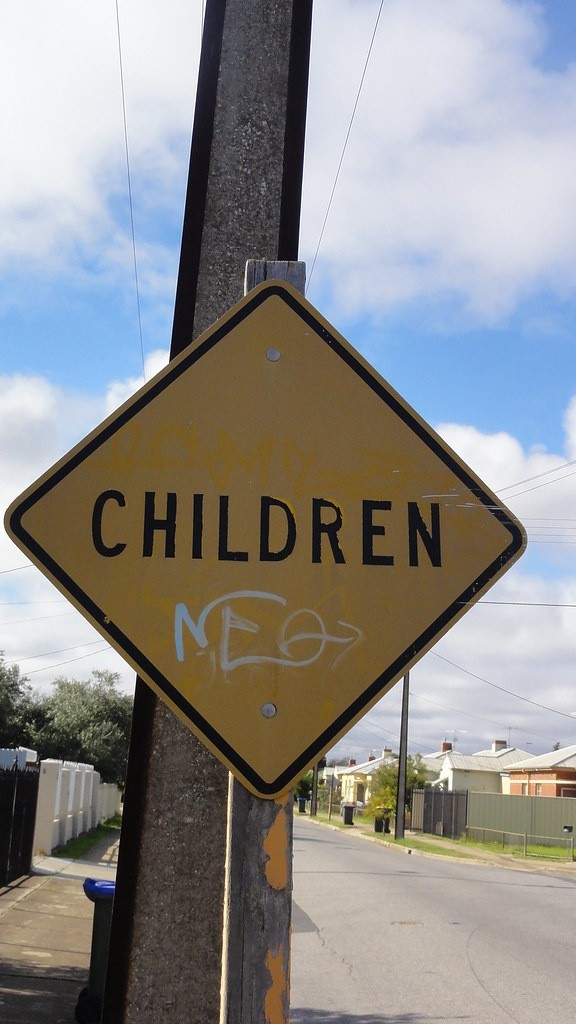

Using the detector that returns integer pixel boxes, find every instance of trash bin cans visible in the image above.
[374,807,394,834]
[343,805,355,825]
[74,877,116,1024]
[297,797,306,813]
